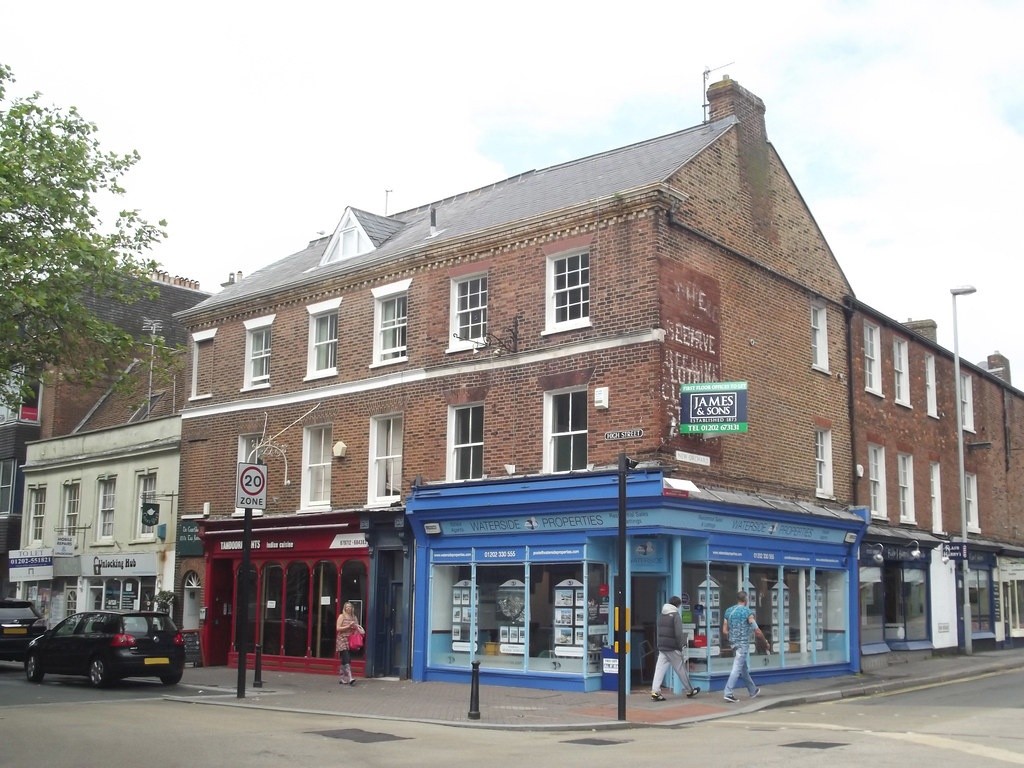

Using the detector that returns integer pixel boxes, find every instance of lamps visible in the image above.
[452,318,521,357]
[933,545,953,565]
[865,541,884,564]
[906,540,922,558]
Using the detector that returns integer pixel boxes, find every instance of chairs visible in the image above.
[91,622,104,633]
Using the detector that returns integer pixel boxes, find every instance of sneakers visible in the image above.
[687,687,700,697]
[724,696,740,703]
[650,692,666,701]
[750,688,760,698]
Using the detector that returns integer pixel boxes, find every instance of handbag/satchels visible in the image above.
[349,629,363,650]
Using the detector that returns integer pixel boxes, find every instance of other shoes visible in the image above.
[350,679,355,685]
[339,680,348,684]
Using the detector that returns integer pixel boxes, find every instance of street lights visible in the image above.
[950,283,979,657]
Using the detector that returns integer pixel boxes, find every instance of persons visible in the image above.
[723,592,770,702]
[336,602,365,685]
[651,596,701,701]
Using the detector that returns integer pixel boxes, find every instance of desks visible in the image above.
[548,648,606,673]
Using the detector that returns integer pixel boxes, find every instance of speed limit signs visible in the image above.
[240,466,266,497]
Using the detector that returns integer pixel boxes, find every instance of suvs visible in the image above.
[24,608,187,690]
[0,597,49,663]
